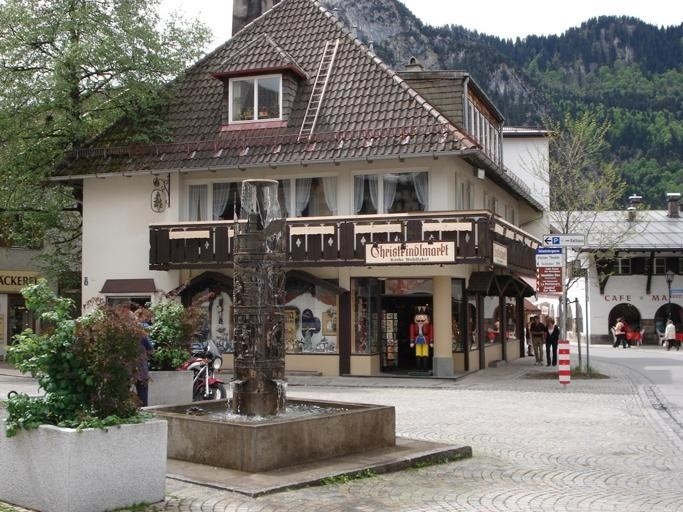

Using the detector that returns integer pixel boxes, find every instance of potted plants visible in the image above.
[0,278,168,512]
[129,299,208,406]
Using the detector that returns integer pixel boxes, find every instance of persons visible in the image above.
[664,319,680,351]
[612,317,629,348]
[526,315,560,366]
[127,309,152,406]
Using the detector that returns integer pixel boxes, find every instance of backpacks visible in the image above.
[620,326,624,332]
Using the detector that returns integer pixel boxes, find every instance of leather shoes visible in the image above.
[546,363,556,367]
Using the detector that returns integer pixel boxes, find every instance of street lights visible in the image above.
[665,269,675,321]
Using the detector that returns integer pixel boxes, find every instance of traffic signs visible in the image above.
[542,233,585,248]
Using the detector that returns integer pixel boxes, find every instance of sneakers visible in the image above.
[527,352,534,356]
[535,361,543,365]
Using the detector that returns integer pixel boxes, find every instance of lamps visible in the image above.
[151,173,170,212]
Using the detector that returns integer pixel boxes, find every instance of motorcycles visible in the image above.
[175,341,228,403]
